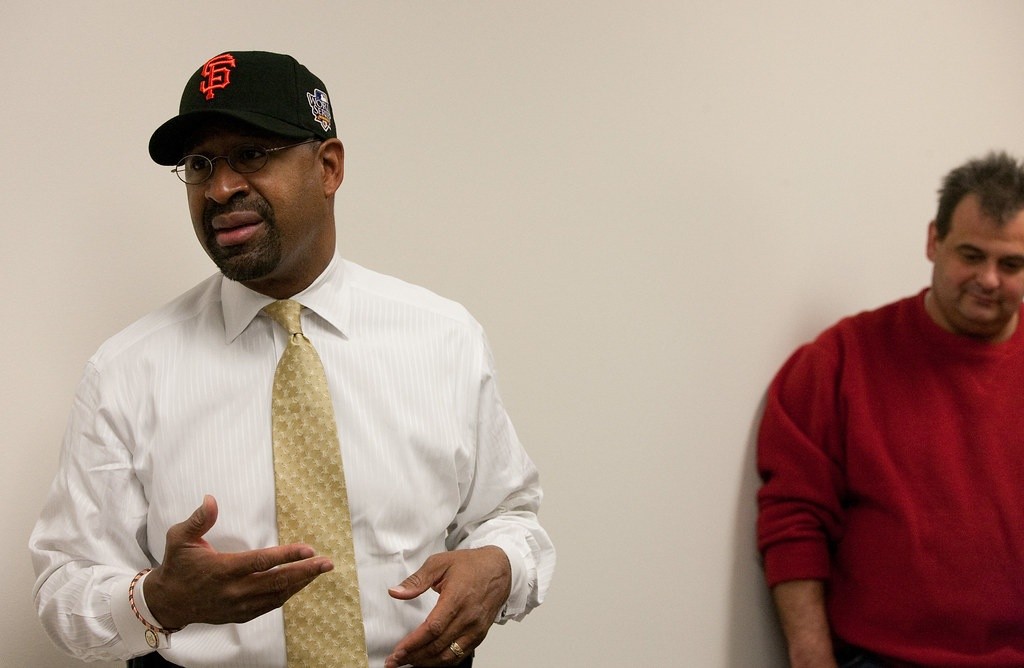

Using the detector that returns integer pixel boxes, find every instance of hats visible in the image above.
[149,51,337,167]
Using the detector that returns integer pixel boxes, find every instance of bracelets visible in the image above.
[129,570,187,635]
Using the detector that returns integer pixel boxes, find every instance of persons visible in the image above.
[27,51,555,668]
[756,154,1024,668]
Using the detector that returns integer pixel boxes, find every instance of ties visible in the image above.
[258,301,369,668]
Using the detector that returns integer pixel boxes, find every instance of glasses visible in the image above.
[171,138,318,186]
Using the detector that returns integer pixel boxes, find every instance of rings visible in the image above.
[447,642,463,658]
